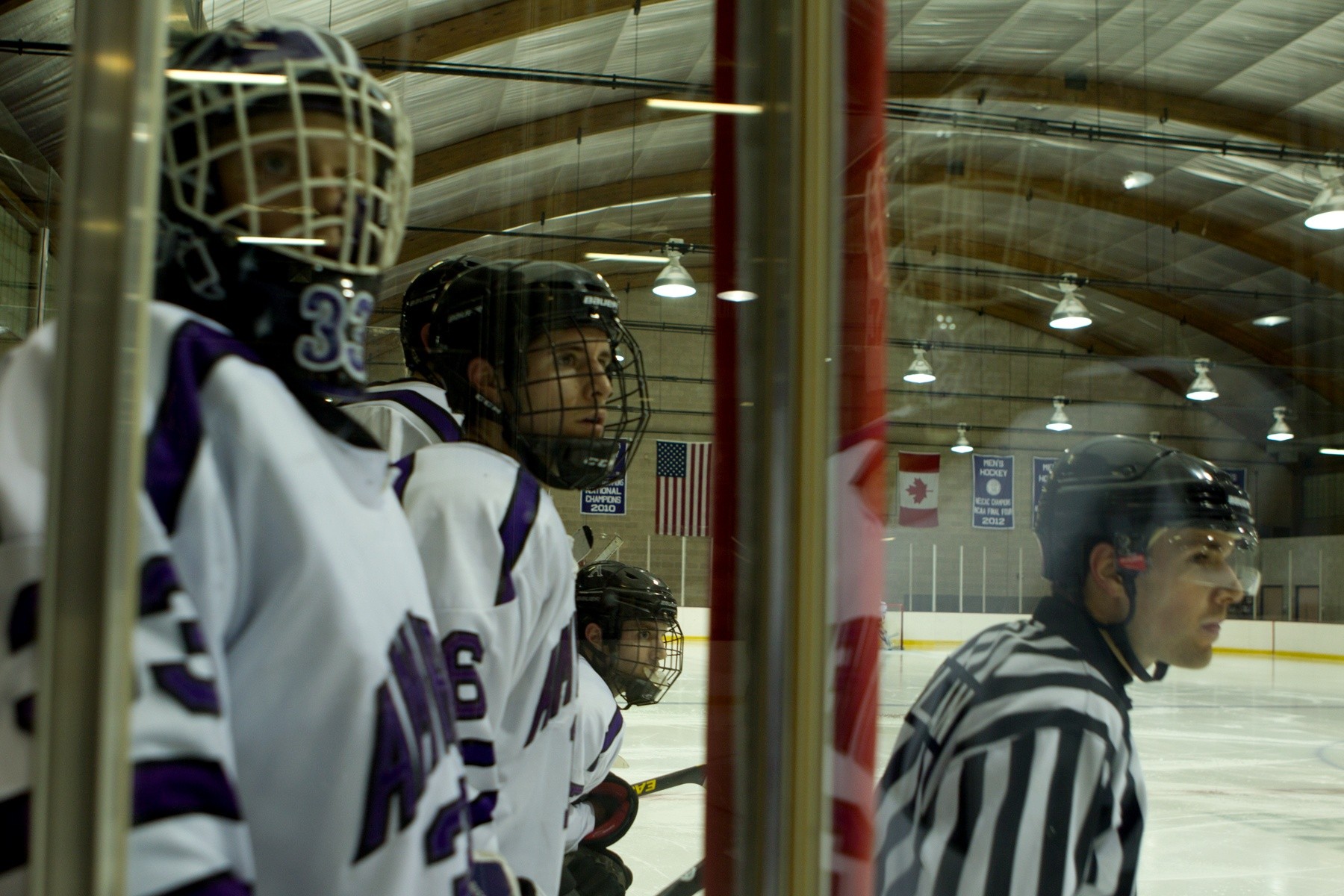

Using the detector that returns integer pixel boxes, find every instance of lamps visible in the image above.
[1046,395,1073,431]
[1266,406,1294,441]
[1305,154,1344,230]
[951,423,973,452]
[903,340,935,383]
[652,237,696,298]
[1185,357,1219,401]
[1049,273,1092,329]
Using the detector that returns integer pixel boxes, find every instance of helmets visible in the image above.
[399,260,485,387]
[574,559,684,710]
[1033,436,1256,579]
[149,17,415,388]
[429,254,652,493]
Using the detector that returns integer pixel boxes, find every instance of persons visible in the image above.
[324,256,686,896]
[871,437,1261,896]
[0,16,473,896]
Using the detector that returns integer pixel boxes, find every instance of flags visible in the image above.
[654,439,712,537]
[897,450,940,529]
